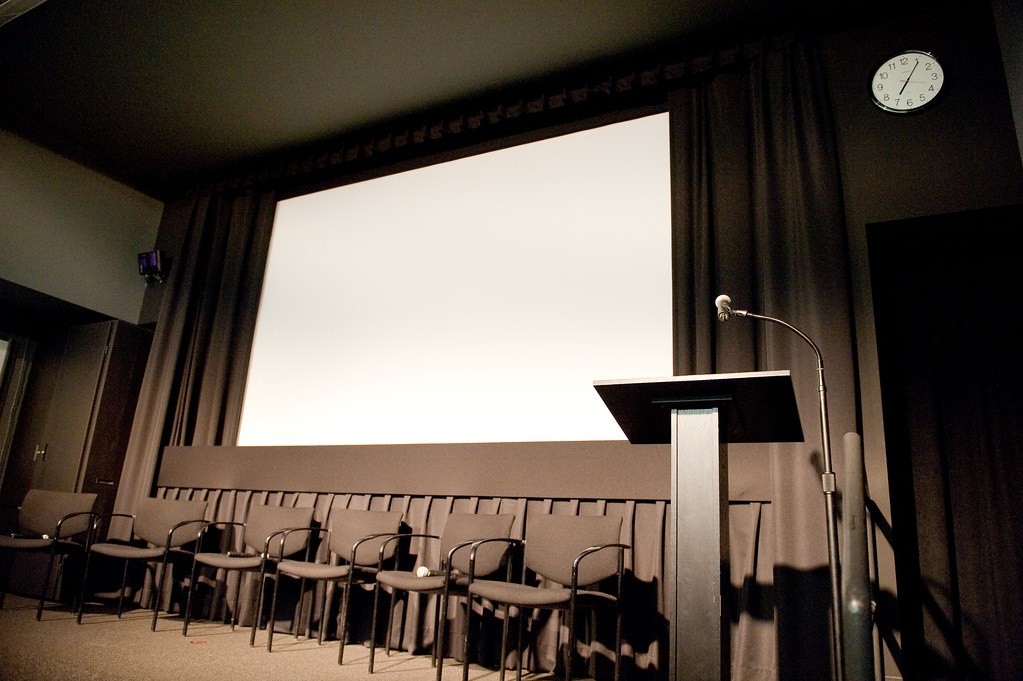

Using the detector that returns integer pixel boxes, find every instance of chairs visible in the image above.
[0,490,98,620]
[366,512,514,680]
[182,504,313,644]
[462,513,630,681]
[76,496,208,630]
[266,507,404,662]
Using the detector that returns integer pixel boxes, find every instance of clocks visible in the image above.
[866,46,948,115]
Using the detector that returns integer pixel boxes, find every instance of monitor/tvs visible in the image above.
[137,250,160,275]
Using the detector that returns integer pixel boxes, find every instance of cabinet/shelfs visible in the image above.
[1,319,154,602]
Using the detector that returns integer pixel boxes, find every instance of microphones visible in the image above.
[715,294,731,321]
